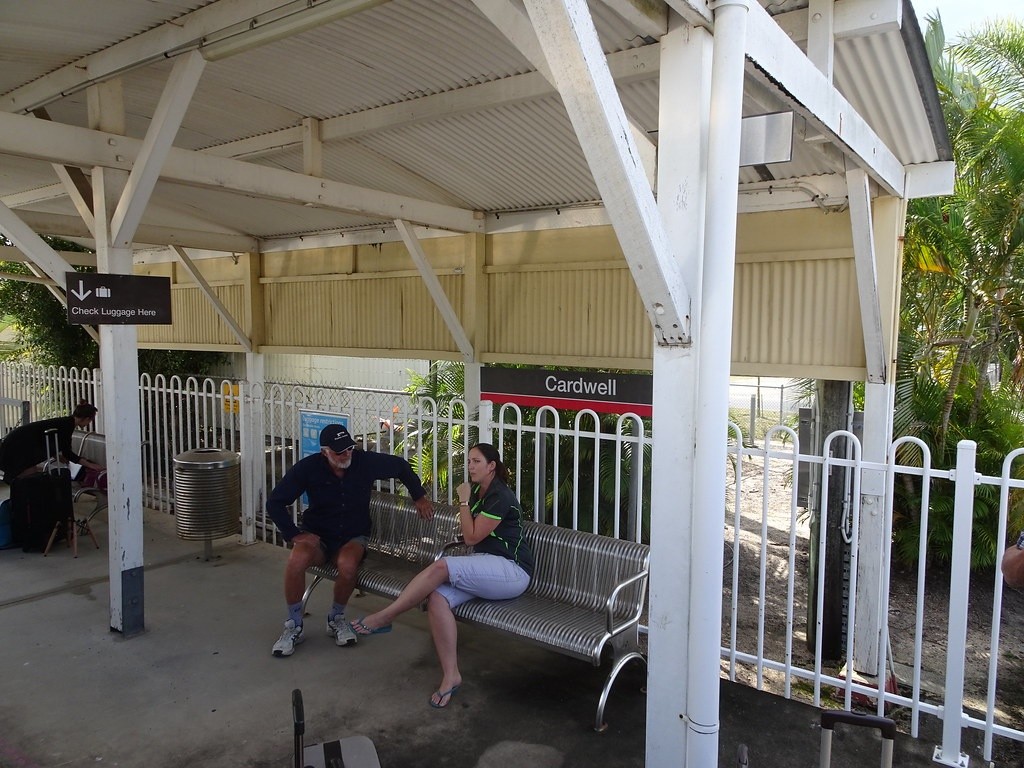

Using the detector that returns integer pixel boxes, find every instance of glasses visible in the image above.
[325,446,354,455]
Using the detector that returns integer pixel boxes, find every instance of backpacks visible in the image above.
[0,499,11,550]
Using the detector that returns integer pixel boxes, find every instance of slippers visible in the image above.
[430,684,459,708]
[348,620,396,637]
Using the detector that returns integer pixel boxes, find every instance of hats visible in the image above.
[319,424,358,453]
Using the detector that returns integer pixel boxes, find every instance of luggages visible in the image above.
[10,428,73,552]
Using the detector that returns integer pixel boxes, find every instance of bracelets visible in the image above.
[459,501,470,507]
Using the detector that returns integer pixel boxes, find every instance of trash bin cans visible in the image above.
[174,448,242,542]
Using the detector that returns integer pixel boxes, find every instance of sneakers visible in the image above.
[272,618,306,656]
[327,614,357,646]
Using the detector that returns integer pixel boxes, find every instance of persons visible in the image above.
[0,403,104,486]
[348,444,532,709]
[265,425,434,659]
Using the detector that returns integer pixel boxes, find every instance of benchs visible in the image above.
[57,429,150,536]
[291,488,651,734]
